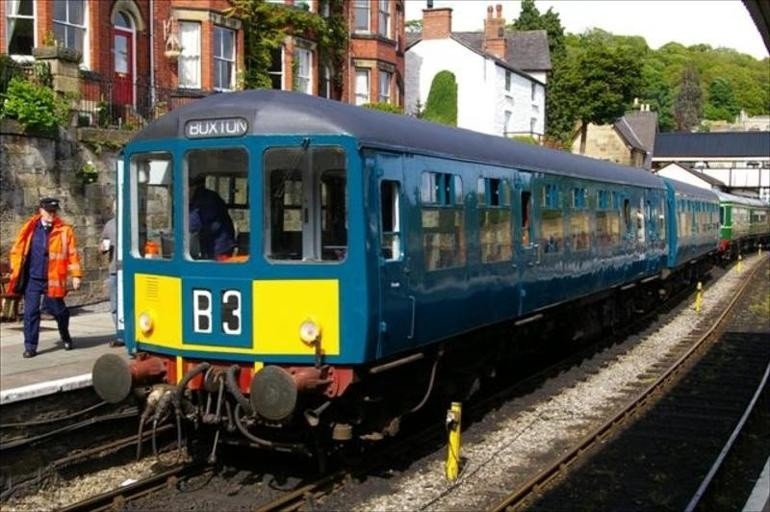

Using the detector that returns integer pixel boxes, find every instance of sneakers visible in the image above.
[24,350,36,357]
[65,341,71,348]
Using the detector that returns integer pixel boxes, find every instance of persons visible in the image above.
[97,199,124,346]
[4,197,82,358]
[189,177,238,259]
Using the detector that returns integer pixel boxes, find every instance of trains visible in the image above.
[92,89,770,474]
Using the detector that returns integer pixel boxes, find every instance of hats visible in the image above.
[40,198,60,211]
